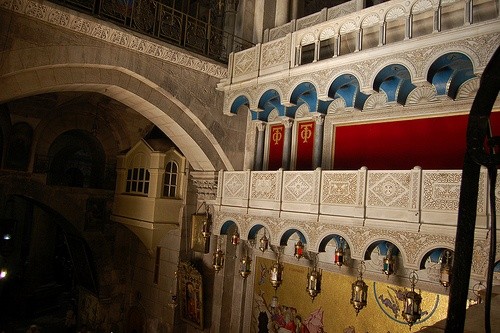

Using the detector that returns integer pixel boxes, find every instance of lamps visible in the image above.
[200,219,488,329]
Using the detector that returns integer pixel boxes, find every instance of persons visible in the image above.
[26,324,40,333]
[77,324,91,333]
[63,304,74,333]
